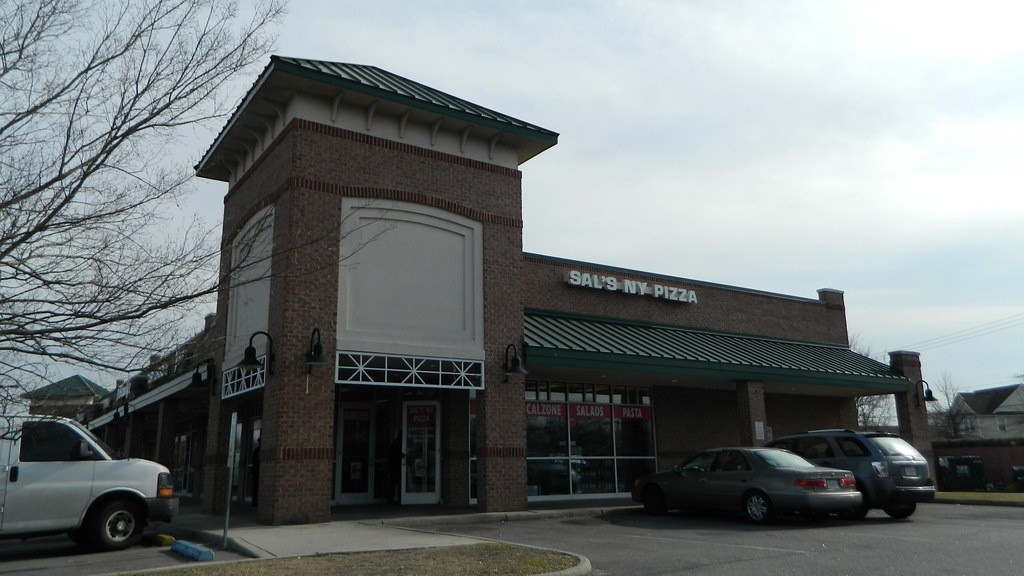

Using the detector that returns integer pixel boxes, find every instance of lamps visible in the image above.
[307,328,329,374]
[235,331,275,375]
[503,344,528,376]
[915,380,938,408]
[186,358,217,394]
[111,401,130,426]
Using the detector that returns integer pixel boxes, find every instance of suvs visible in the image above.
[762,427,934,518]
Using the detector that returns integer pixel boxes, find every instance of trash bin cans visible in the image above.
[938,454,984,489]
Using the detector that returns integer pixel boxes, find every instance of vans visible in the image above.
[0,412,180,551]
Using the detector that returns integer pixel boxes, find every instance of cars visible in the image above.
[630,448,864,526]
